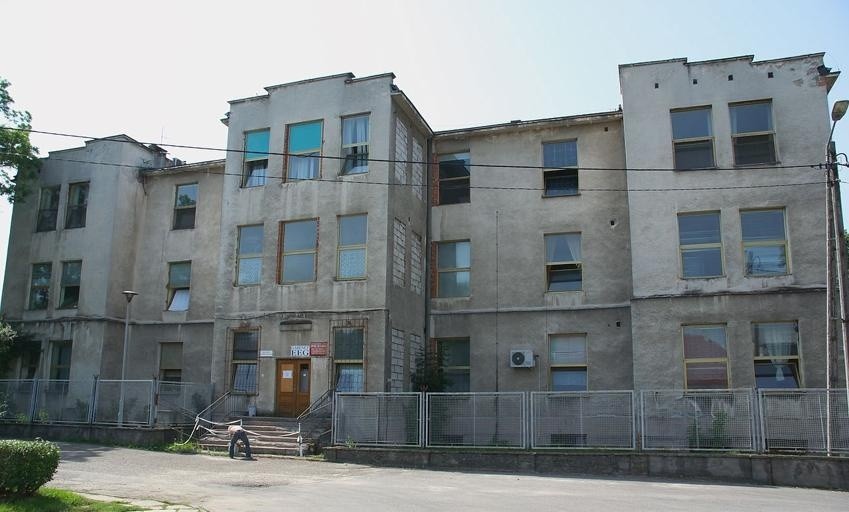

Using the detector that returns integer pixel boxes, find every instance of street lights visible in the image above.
[117,290,140,428]
[818,97,849,457]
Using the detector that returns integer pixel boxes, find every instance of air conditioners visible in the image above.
[173,157,183,165]
[509,349,535,367]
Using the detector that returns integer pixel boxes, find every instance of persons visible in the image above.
[227,425,260,459]
